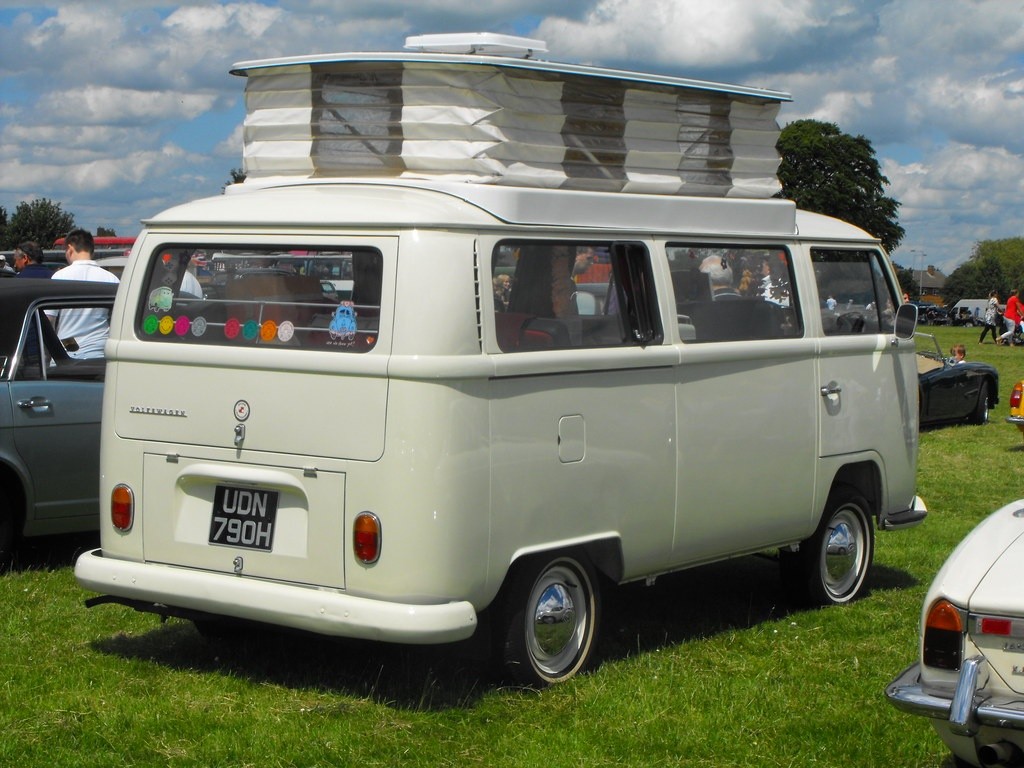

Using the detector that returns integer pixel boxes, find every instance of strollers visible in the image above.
[999,312,1023,346]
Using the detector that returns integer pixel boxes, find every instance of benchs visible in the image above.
[491,312,574,350]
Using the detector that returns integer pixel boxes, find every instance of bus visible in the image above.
[212,251,354,302]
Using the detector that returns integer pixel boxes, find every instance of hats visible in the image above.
[0,254,6,260]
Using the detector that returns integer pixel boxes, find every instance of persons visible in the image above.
[0,254,14,272]
[866,301,876,310]
[978,290,1004,343]
[494,274,510,300]
[700,255,743,302]
[872,289,910,324]
[996,289,1024,347]
[570,246,596,283]
[178,270,202,299]
[826,294,837,312]
[951,344,965,363]
[757,255,789,307]
[43,229,120,382]
[926,306,973,327]
[14,241,57,365]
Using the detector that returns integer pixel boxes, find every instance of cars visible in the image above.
[914,331,1000,426]
[0,278,210,576]
[882,497,1024,768]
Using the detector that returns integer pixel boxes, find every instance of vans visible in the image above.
[946,300,990,326]
[75,32,929,694]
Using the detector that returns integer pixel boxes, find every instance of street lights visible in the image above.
[911,249,924,295]
[912,254,928,277]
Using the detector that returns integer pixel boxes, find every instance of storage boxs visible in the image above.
[219,272,323,336]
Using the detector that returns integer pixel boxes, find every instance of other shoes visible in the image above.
[996,337,1002,345]
[978,341,983,345]
[1010,343,1015,347]
